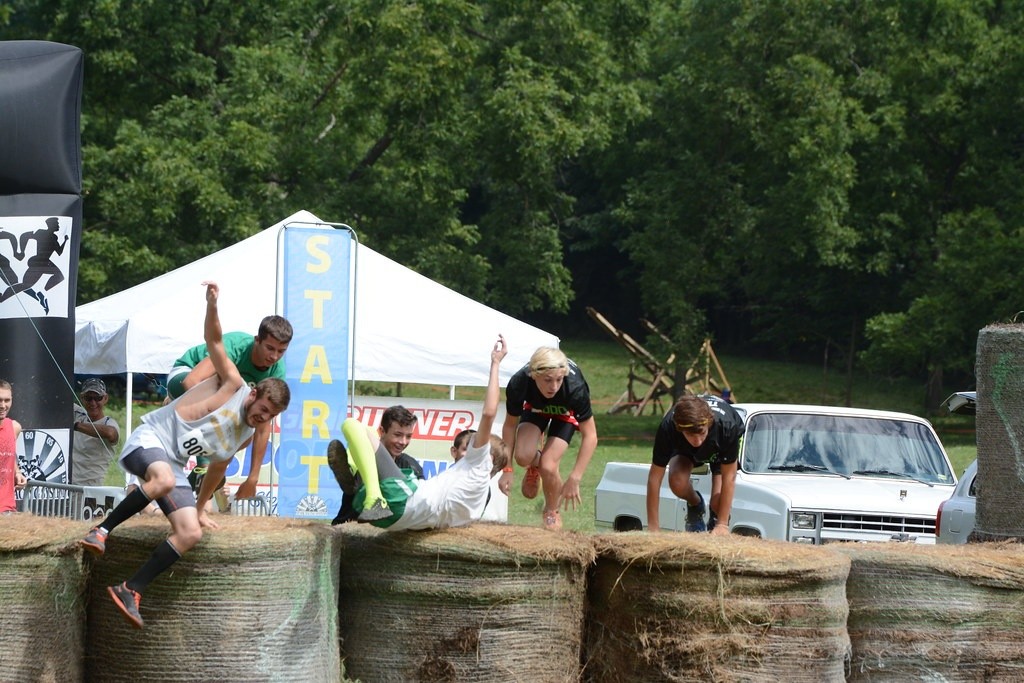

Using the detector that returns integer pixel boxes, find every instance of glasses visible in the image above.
[83,394,104,402]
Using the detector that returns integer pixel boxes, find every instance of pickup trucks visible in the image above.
[591,404,957,552]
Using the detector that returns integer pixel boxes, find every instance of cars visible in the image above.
[935,392,980,545]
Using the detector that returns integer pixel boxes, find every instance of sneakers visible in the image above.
[684,491,706,533]
[358,495,392,521]
[81,526,108,556]
[522,450,543,498]
[541,506,561,531]
[107,581,146,630]
[703,505,732,530]
[327,440,352,490]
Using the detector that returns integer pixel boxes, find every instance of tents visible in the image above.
[72,207,563,488]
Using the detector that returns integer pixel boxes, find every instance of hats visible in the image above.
[80,379,106,396]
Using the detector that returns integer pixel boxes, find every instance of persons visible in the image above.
[498,346,597,530]
[646,392,745,535]
[79,279,291,626]
[0,378,26,516]
[163,313,292,506]
[376,403,425,479]
[72,376,119,521]
[447,429,477,470]
[329,328,507,532]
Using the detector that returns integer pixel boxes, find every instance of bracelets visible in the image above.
[503,468,513,472]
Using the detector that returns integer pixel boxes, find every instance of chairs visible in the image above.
[232,496,270,516]
